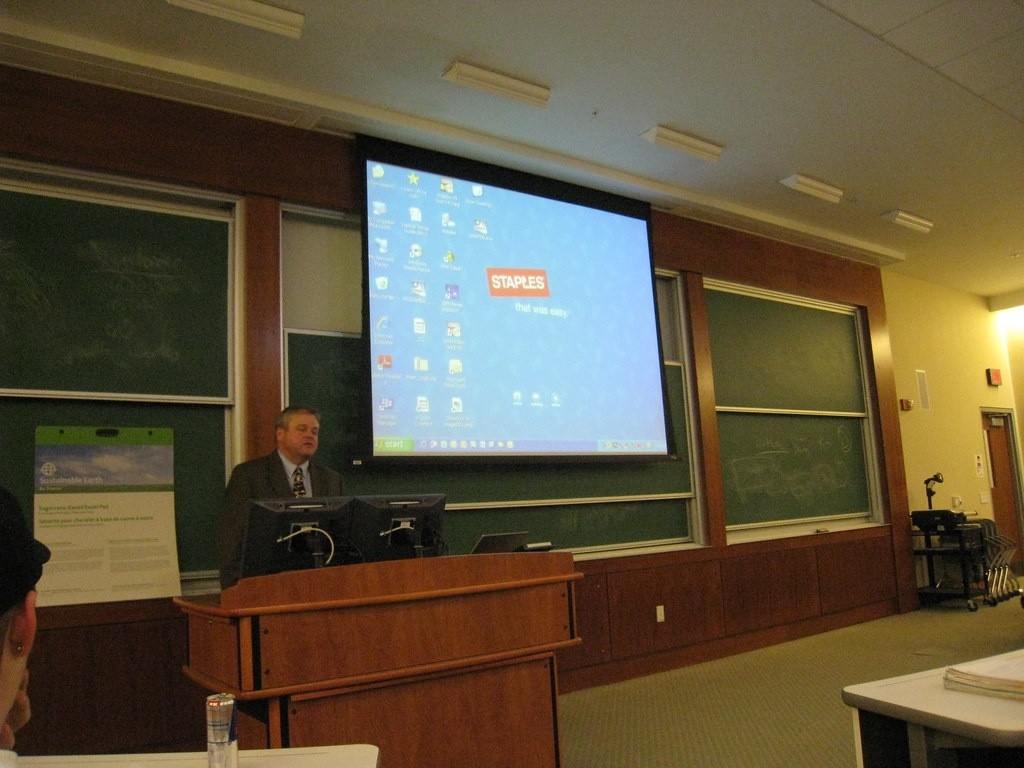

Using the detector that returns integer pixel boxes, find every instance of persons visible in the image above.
[0,487,51,768]
[216,404,354,592]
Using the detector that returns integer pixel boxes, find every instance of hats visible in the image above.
[0,486,51,615]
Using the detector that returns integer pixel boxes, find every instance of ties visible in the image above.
[293,467,307,499]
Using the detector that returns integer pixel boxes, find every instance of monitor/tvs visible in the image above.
[239,496,353,580]
[346,493,446,565]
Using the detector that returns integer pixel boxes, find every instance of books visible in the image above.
[944,649,1024,700]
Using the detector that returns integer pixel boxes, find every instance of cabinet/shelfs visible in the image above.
[912,524,990,611]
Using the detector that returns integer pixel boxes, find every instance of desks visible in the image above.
[19,741,382,768]
[842,648,1024,768]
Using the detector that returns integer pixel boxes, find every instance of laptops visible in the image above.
[468,531,531,554]
